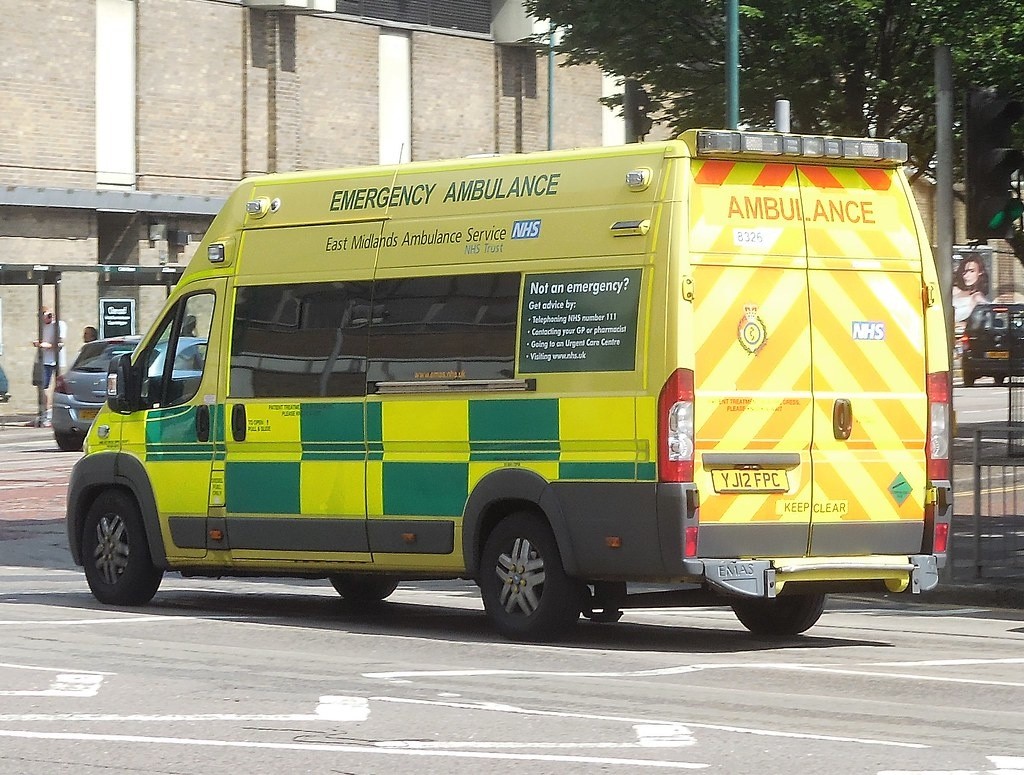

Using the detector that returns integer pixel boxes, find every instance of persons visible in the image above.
[82,325,99,342]
[26,306,68,429]
[182,316,200,338]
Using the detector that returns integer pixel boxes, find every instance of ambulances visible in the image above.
[65,98,955,644]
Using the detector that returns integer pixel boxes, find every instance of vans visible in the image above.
[961,303,1024,387]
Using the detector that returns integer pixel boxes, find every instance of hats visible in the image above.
[42,305,52,316]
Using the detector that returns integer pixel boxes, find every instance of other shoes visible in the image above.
[44,421,52,427]
[24,421,35,427]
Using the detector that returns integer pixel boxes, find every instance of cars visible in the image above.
[51,335,211,451]
